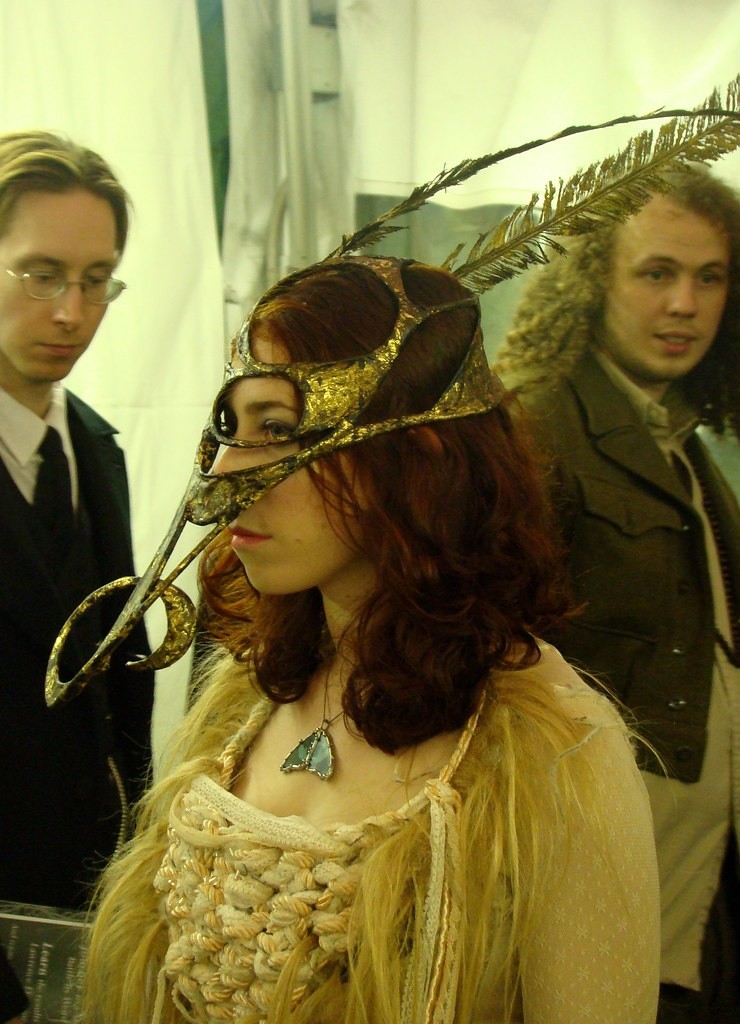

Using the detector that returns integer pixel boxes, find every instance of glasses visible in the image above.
[0,261,127,304]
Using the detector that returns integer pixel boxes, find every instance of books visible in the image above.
[0,899,102,1024]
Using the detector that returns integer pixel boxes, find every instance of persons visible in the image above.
[486,154,740,1024]
[0,129,155,1024]
[79,254,660,1024]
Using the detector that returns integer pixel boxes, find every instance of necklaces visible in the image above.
[278,657,370,781]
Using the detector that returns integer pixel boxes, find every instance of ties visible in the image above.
[32,426,74,570]
